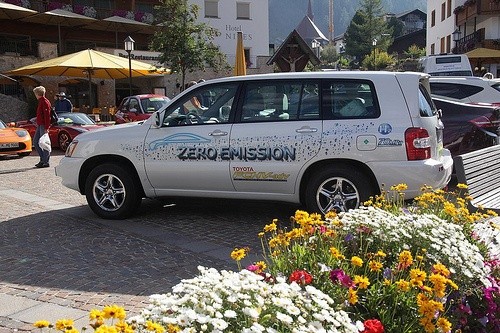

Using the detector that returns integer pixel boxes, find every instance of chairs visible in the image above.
[73,105,115,121]
[246,93,268,120]
[275,94,290,119]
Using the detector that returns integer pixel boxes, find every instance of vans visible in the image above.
[423,53,473,77]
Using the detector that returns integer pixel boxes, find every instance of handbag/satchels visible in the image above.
[38,133,51,152]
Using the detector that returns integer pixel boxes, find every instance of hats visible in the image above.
[58,92,67,96]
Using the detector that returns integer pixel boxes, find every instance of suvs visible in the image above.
[54,72,454,222]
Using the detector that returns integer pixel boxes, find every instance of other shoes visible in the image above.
[35,162,49,168]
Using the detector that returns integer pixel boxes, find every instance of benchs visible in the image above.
[453,145,500,216]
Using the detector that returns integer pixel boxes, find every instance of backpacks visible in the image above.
[40,99,58,123]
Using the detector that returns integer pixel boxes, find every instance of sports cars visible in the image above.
[0,119,32,156]
[15,112,113,153]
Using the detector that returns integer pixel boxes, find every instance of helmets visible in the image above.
[481,67,484,71]
[475,67,478,71]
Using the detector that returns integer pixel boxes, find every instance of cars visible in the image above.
[112,94,180,125]
[290,76,500,191]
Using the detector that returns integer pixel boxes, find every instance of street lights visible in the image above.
[339,43,342,71]
[372,38,378,71]
[123,36,136,96]
[316,42,321,67]
[311,39,318,72]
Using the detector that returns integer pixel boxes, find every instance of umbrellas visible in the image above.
[0,49,177,109]
[234,32,246,76]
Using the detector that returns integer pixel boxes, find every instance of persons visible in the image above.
[180,79,212,115]
[53,92,73,113]
[33,86,52,168]
[72,104,118,121]
